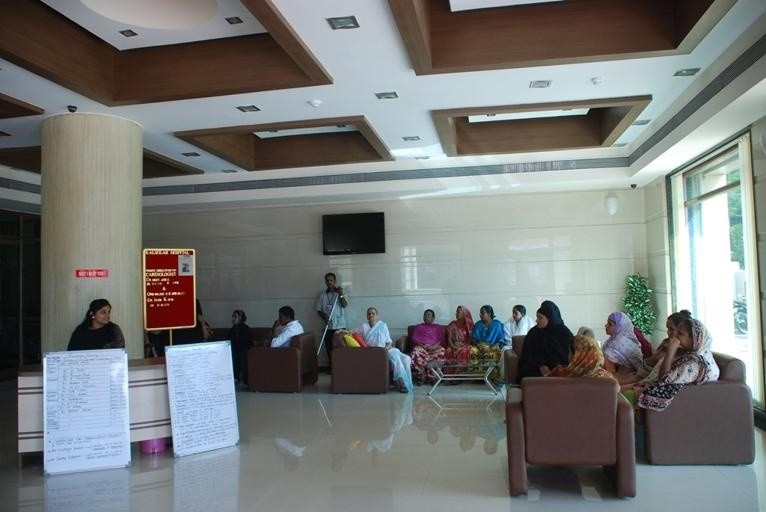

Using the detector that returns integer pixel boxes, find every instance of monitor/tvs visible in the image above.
[321,212,385,256]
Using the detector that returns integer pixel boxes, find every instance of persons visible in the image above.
[518,302,612,379]
[601,310,719,417]
[410,301,533,384]
[266,306,305,348]
[335,307,414,392]
[68,299,125,349]
[412,392,507,454]
[315,272,348,373]
[146,297,250,386]
[265,432,394,474]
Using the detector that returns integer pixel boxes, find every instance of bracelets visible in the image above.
[339,295,344,299]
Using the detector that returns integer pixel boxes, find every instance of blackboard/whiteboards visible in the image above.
[165,340,240,458]
[42,348,131,476]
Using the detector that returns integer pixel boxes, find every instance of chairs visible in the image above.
[330,329,399,394]
[506,376,637,497]
[247,332,318,392]
[504,335,526,393]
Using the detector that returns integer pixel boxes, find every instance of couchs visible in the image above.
[396,324,448,381]
[154,328,264,381]
[643,353,755,465]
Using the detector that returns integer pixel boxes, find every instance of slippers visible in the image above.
[399,386,408,393]
